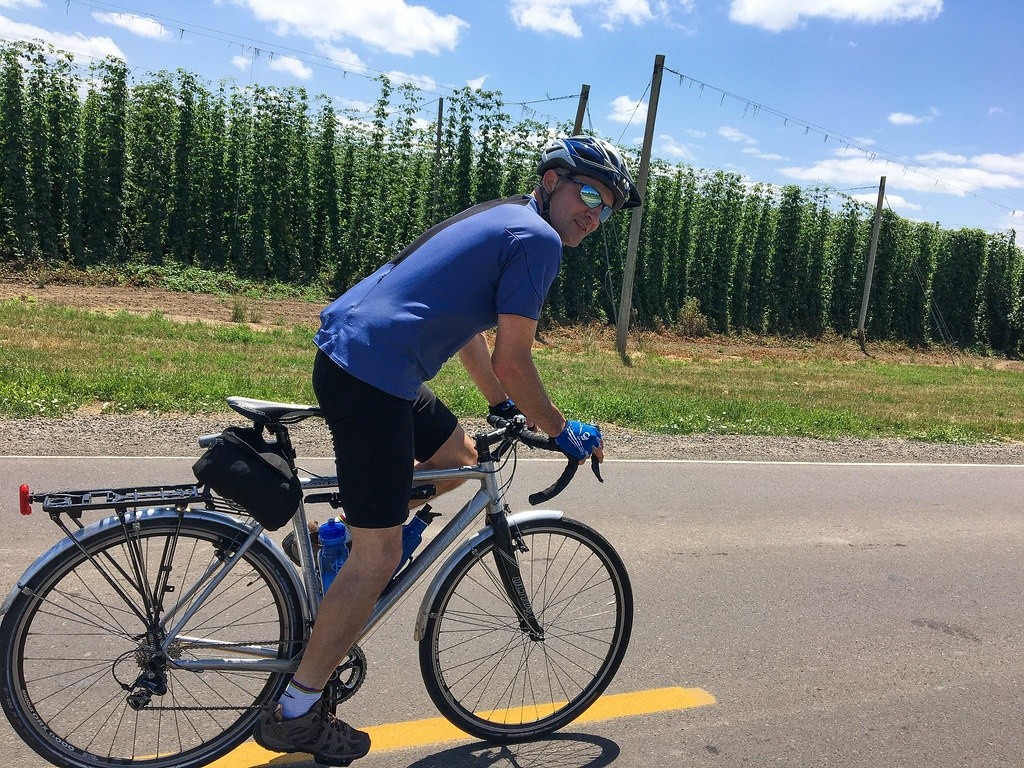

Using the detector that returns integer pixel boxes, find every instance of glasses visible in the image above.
[556,173,613,224]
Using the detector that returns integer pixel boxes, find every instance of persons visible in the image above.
[251,134,642,761]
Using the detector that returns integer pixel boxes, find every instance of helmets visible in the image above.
[536,136,642,211]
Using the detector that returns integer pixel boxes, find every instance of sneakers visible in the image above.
[252,698,371,760]
[281,520,353,570]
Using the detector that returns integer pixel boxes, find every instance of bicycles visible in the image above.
[0,408,633,768]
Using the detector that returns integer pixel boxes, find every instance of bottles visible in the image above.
[317,518,349,597]
[387,504,442,582]
